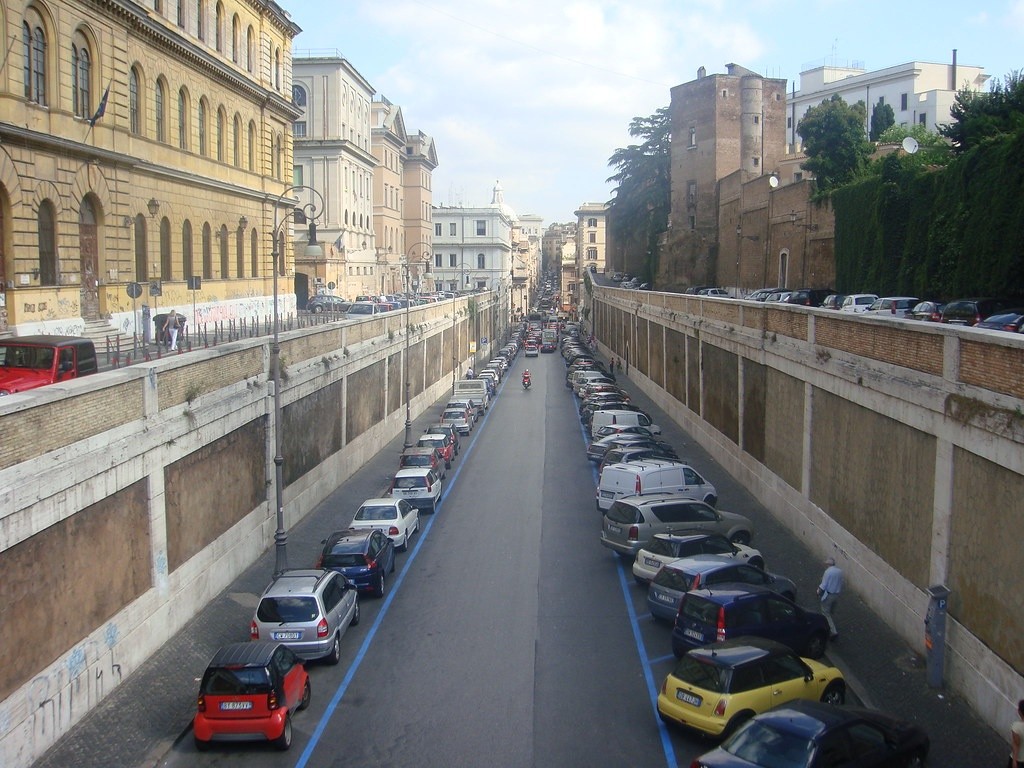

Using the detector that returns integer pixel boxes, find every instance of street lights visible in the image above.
[271,185,328,582]
[472,270,488,379]
[453,262,472,395]
[402,242,434,452]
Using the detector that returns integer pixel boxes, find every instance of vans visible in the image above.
[596,456,718,517]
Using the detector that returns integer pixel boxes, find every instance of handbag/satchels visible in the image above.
[174,316,179,328]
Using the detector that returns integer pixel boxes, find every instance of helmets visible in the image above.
[525,369,529,372]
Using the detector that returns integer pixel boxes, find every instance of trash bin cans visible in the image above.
[473,374,477,380]
[153,313,187,345]
[591,267,596,273]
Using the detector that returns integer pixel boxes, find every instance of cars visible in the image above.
[904,300,947,322]
[818,294,847,310]
[745,287,794,305]
[0,334,98,397]
[972,307,1024,334]
[388,468,442,514]
[836,293,879,313]
[646,553,799,626]
[437,275,682,483]
[399,446,446,480]
[316,529,395,597]
[685,286,730,298]
[632,529,765,589]
[344,301,381,320]
[348,498,419,552]
[423,422,462,457]
[356,288,480,313]
[863,296,920,319]
[688,697,933,768]
[415,433,455,470]
[656,634,846,745]
[191,639,312,752]
[611,271,651,291]
[670,578,830,662]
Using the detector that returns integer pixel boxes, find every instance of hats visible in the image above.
[825,559,834,564]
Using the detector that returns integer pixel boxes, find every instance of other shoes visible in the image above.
[829,633,838,642]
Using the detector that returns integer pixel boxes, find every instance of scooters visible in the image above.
[522,372,531,390]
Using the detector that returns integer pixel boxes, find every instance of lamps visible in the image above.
[737,225,759,242]
[216,216,248,238]
[789,209,819,232]
[375,246,393,257]
[350,241,366,254]
[125,197,160,228]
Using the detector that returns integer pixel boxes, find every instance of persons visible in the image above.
[586,334,599,354]
[1009,699,1024,768]
[521,368,531,386]
[465,366,473,380]
[816,558,844,636]
[580,318,587,336]
[610,356,615,374]
[616,357,623,374]
[162,310,181,351]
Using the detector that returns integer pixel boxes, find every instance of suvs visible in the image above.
[250,568,360,666]
[306,294,352,314]
[939,296,1008,326]
[788,287,831,307]
[599,492,755,560]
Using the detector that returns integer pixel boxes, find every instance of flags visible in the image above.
[90,84,110,127]
